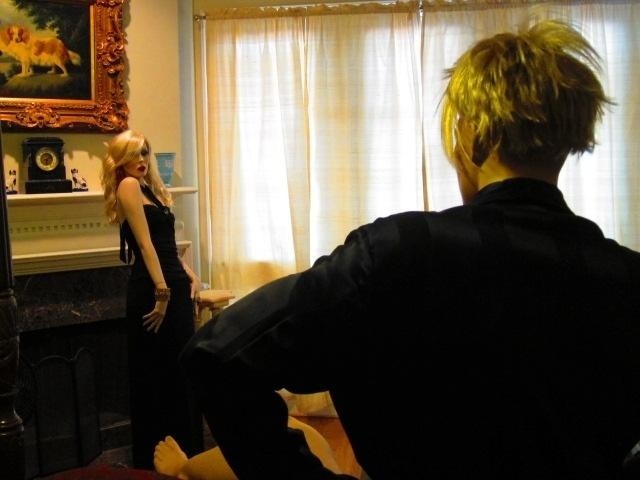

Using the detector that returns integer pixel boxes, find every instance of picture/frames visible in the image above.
[0,0,129,134]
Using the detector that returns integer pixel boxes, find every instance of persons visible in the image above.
[102,129,201,470]
[154,415,360,480]
[180,4,640,480]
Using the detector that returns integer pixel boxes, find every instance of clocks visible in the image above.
[22,136,72,194]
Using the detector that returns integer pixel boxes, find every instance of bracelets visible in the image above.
[154,288,171,301]
